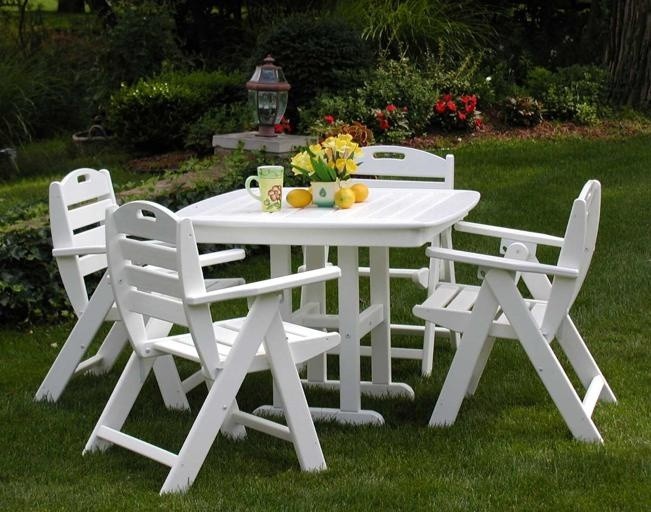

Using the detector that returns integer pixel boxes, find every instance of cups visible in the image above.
[245,164,285,213]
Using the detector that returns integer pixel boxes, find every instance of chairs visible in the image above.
[33,166,246,406]
[298,144,454,379]
[83,198,342,495]
[411,180,617,447]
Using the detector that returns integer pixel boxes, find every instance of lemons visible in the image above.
[334,188,356,209]
[286,189,312,209]
[351,183,368,202]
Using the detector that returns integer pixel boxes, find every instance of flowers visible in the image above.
[288,131,365,182]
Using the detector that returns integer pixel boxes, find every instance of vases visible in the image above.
[310,180,345,206]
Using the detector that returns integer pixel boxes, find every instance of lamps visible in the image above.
[248,54,292,136]
